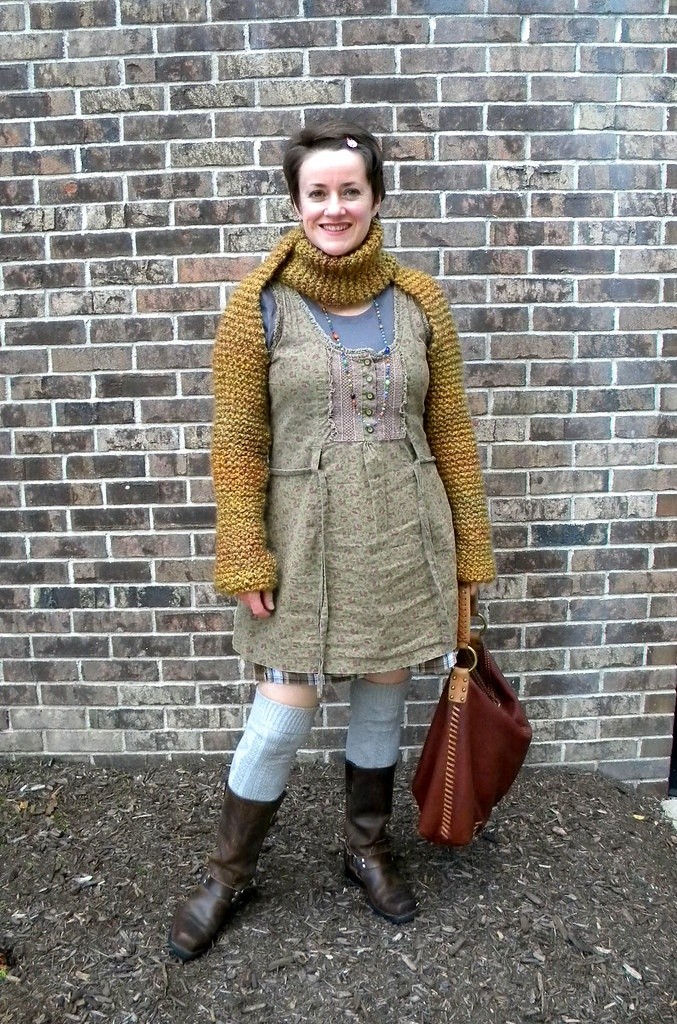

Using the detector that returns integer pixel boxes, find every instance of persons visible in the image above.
[167,117,498,959]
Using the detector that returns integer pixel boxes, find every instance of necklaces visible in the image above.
[322,299,391,427]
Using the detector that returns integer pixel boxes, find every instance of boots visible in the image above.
[169,781,287,961]
[344,758,420,924]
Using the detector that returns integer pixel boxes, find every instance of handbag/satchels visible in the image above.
[413,583,531,848]
